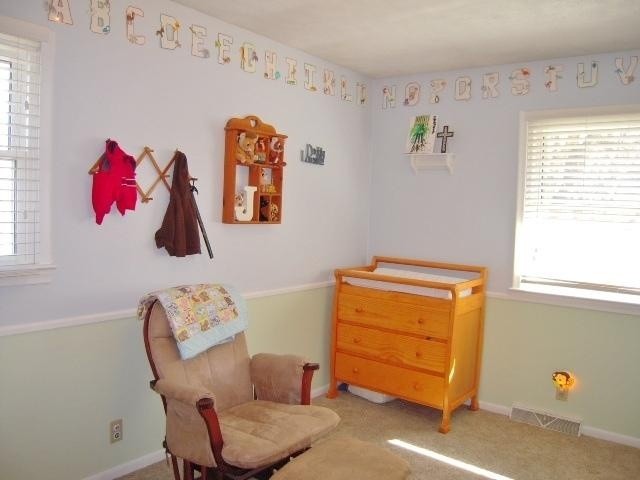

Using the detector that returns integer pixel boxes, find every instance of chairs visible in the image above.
[143,287,341,479]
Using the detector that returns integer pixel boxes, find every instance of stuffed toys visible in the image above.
[234,131,287,224]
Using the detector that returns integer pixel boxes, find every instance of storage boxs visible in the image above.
[345,383,398,404]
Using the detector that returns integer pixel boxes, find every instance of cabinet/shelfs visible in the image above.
[222,116,288,225]
[326,255,485,434]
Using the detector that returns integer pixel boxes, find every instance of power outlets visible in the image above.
[108,417,123,444]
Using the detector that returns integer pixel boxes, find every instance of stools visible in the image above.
[269,433,413,480]
